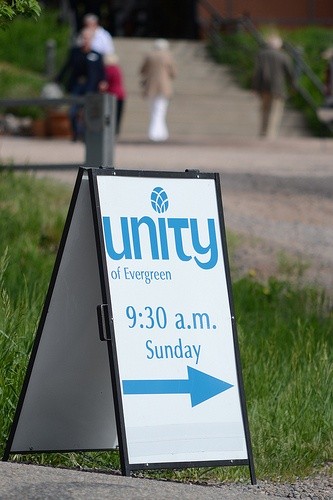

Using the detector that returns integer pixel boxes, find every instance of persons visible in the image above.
[99,53,125,138]
[140,39,173,143]
[77,14,113,56]
[256,30,294,141]
[54,29,108,143]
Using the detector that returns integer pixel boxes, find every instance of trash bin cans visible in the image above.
[83,90,118,168]
